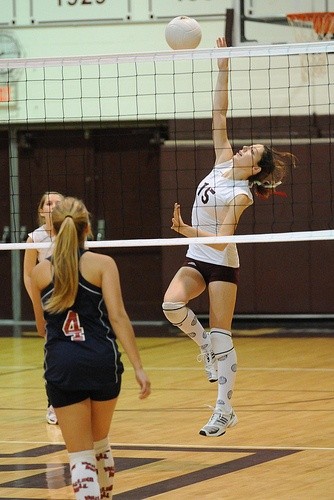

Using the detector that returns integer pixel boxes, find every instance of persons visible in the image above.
[23,191,68,424]
[161,36,296,438]
[36,197,151,500]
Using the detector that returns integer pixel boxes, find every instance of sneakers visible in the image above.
[199,405,238,437]
[197,332,218,383]
[47,404,58,424]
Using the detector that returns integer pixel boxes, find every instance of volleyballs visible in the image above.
[164,16,202,50]
